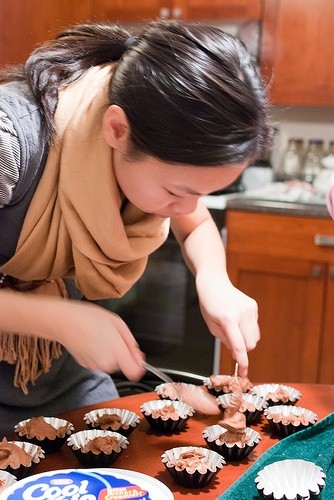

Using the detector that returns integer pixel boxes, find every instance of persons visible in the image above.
[0,22,280,435]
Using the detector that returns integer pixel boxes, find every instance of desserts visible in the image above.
[0,374,319,490]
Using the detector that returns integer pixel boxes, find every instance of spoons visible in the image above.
[226,357,243,418]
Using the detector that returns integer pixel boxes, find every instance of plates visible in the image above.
[0,468,177,500]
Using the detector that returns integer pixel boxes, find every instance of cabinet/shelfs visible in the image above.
[221,208,334,384]
[0,0,334,105]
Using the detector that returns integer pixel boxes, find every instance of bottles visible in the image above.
[140,359,221,417]
[278,138,334,182]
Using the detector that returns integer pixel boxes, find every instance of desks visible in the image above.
[7,380,334,500]
